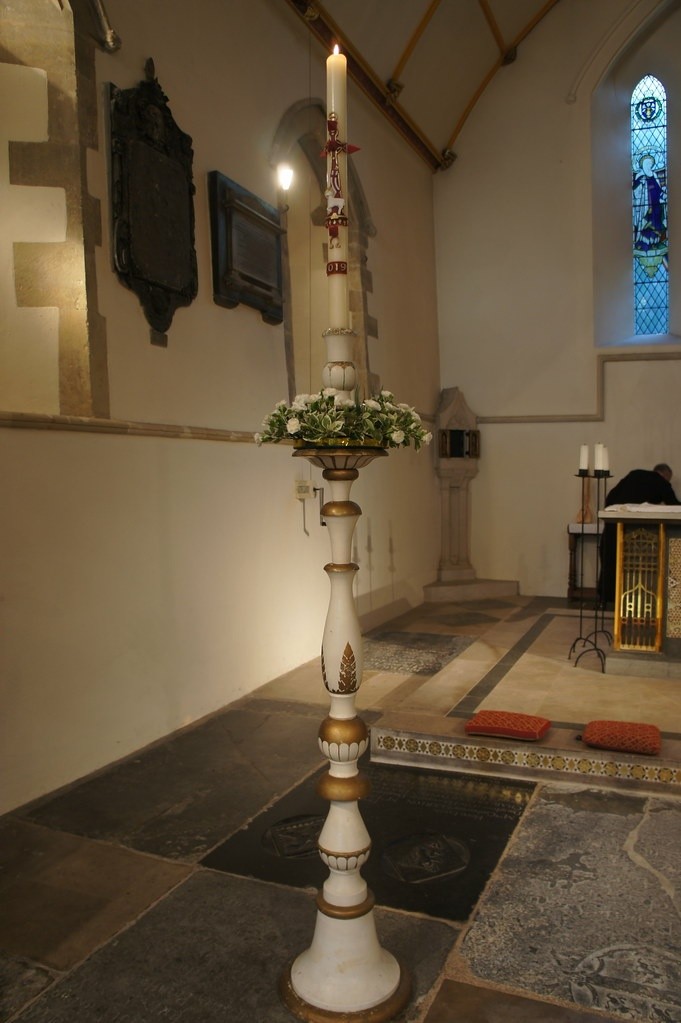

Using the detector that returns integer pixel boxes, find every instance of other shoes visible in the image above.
[596,600,606,610]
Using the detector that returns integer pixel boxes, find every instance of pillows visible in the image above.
[464,709,551,740]
[581,720,661,754]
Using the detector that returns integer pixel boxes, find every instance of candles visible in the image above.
[324,43,351,329]
[579,443,588,469]
[594,442,609,471]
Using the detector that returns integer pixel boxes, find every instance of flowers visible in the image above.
[252,391,433,453]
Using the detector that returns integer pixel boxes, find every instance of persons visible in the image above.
[598,464,681,602]
